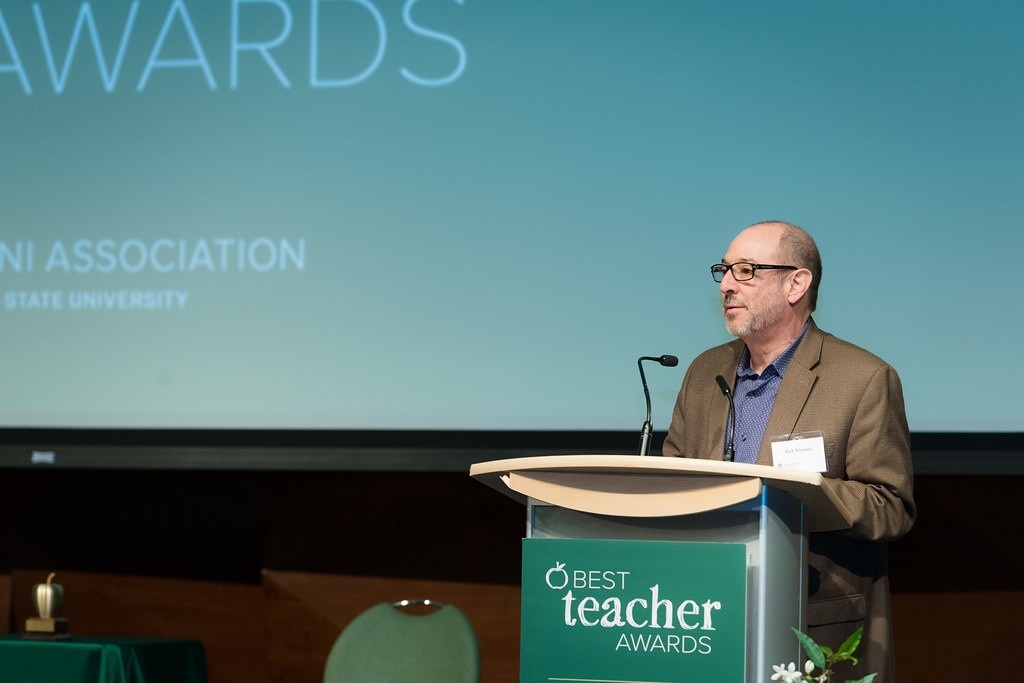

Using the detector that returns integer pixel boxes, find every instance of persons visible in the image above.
[663,221,916,683]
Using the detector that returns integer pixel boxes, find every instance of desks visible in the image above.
[0,634,209,683]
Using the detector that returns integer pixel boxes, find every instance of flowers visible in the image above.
[770,626,879,683]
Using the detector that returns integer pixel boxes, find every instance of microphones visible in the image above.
[715,374,735,461]
[637,355,679,456]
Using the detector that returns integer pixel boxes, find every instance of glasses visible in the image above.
[710,262,798,283]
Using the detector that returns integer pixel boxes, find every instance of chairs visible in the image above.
[324,598,481,683]
